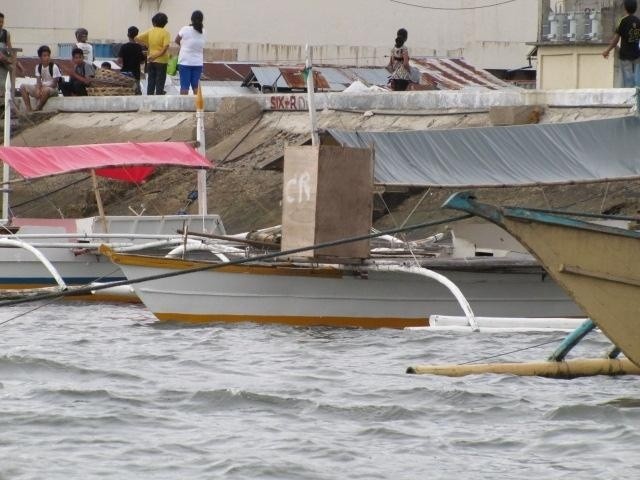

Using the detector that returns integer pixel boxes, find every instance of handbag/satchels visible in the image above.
[165,50,179,75]
[386,62,394,73]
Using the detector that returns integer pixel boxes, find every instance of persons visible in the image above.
[0,12,26,121]
[19,45,60,112]
[117,26,147,95]
[59,48,96,96]
[174,9,206,95]
[389,28,412,91]
[601,0,640,88]
[73,28,94,65]
[133,11,171,95]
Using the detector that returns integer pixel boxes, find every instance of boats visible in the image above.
[439,188,640,368]
[99,242,589,329]
[0,214,227,305]
